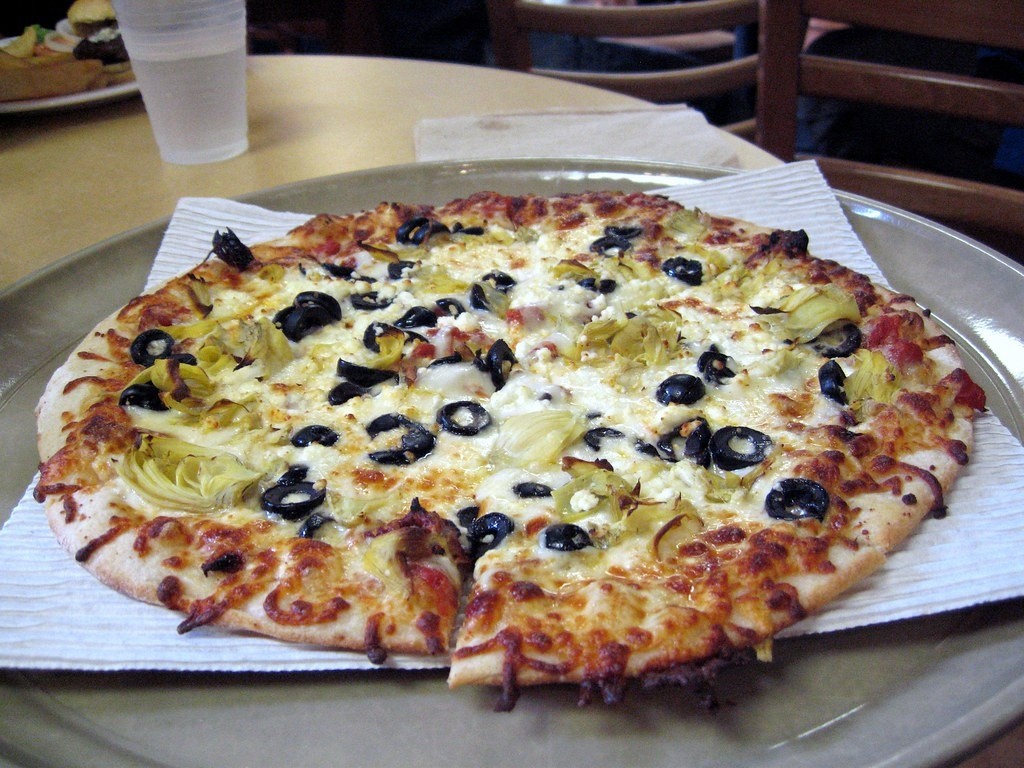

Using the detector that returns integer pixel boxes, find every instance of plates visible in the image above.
[0,37,139,112]
[0,158,1024,768]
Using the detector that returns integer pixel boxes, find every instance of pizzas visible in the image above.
[33,188,989,712]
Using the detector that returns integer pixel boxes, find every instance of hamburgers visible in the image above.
[68,0,137,85]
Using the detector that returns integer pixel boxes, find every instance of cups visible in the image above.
[113,0,250,164]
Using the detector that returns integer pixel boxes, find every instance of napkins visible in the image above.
[411,102,742,170]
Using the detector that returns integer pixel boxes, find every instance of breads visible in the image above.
[0,48,104,105]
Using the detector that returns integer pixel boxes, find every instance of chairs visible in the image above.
[760,0,1024,233]
[485,0,810,144]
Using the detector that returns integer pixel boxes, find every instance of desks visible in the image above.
[0,54,1024,768]
